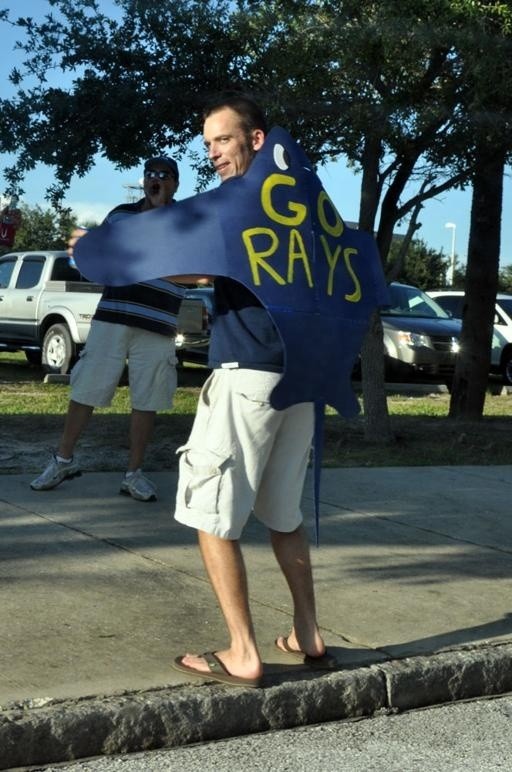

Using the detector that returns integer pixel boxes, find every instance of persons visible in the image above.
[63,96,391,687]
[31,156,201,502]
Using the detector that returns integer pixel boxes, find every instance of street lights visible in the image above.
[445,223,456,285]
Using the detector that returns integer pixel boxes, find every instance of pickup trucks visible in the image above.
[0,250,210,379]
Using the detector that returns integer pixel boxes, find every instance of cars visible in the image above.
[381,283,511,387]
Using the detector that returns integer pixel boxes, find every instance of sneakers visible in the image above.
[120,470,158,501]
[31,453,81,491]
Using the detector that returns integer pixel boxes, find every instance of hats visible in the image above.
[144,157,178,175]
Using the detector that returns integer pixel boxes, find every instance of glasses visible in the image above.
[145,171,176,180]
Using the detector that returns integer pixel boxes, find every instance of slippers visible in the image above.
[173,651,263,687]
[274,635,336,669]
